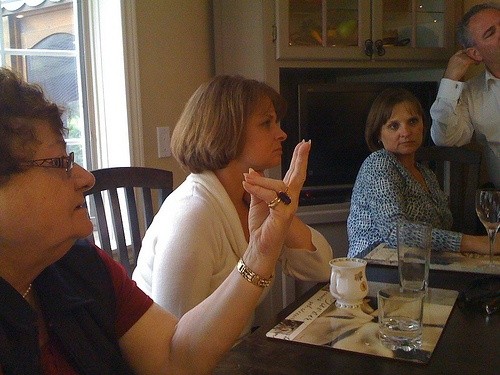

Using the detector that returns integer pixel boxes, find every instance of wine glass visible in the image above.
[475,189,500,274]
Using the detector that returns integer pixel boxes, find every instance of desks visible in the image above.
[210,241,500,375]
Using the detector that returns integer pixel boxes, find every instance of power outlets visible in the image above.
[157,126,171,159]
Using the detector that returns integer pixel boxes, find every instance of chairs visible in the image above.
[79,167,174,278]
[415,147,482,227]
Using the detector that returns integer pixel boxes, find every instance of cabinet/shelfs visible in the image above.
[255,210,350,325]
[212,0,463,61]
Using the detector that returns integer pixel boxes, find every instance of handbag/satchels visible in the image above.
[459,273,500,316]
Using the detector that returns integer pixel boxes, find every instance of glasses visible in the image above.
[0,153,75,177]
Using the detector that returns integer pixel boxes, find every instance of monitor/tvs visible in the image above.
[298,82,439,191]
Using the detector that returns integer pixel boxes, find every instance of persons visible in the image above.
[430,3,500,189]
[347,86,500,259]
[130,72,334,349]
[0,64,312,375]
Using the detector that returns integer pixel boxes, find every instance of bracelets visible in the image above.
[238,257,273,288]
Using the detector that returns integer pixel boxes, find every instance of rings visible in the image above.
[266,191,291,208]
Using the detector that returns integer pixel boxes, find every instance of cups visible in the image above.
[396,221,431,298]
[376,287,423,350]
[328,258,369,309]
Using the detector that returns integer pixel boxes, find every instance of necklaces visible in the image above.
[22,283,32,298]
